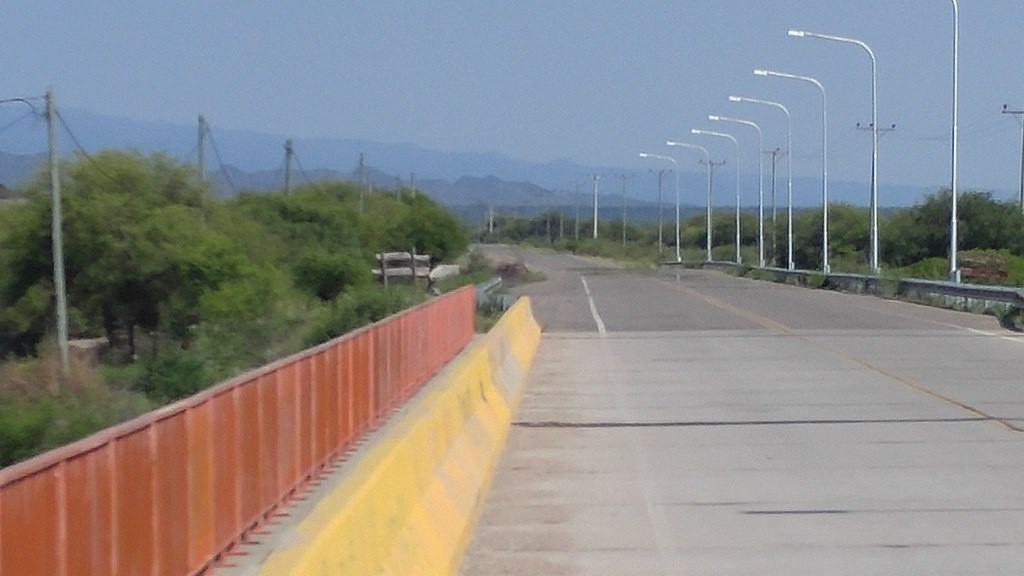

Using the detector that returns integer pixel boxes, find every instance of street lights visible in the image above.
[727,96,794,273]
[638,152,681,262]
[708,115,767,266]
[754,70,831,274]
[667,140,714,263]
[785,30,879,275]
[691,129,743,263]
